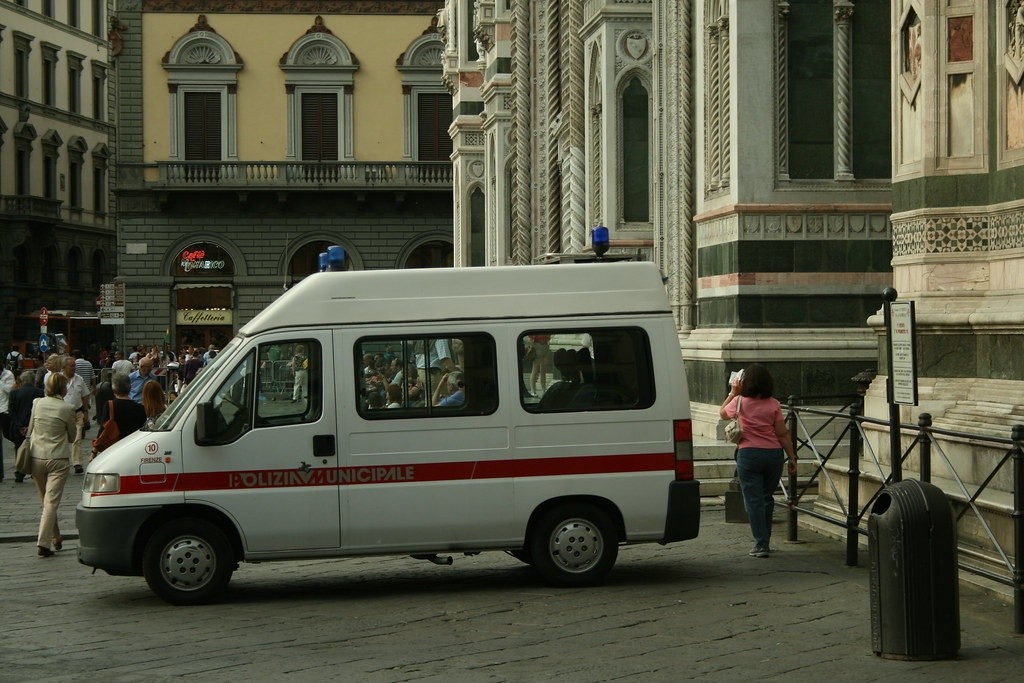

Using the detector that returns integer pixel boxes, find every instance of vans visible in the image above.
[75,227,702,607]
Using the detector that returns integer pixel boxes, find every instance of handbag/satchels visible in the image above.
[302,359,308,369]
[174,383,178,392]
[92,400,119,452]
[180,384,188,394]
[524,345,536,360]
[725,394,743,444]
[16,438,30,474]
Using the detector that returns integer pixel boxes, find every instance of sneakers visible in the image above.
[749,545,769,557]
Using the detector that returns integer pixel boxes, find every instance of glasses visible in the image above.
[143,364,154,369]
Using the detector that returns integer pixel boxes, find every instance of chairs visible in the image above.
[535,346,629,410]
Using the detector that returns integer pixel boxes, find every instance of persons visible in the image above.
[719,364,797,557]
[0,334,551,557]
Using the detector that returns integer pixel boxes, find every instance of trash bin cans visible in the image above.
[154,368,170,391]
[868,477,962,661]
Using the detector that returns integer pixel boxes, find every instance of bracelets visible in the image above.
[728,393,736,396]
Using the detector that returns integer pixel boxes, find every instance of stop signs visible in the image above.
[39,307,48,326]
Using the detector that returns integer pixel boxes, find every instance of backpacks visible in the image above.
[10,352,21,368]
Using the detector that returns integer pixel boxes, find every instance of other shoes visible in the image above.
[15,470,26,482]
[292,400,297,403]
[85,421,91,430]
[53,535,63,550]
[531,393,539,397]
[74,464,84,473]
[38,546,54,555]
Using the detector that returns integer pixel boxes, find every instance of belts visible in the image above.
[418,367,442,370]
[76,406,84,413]
